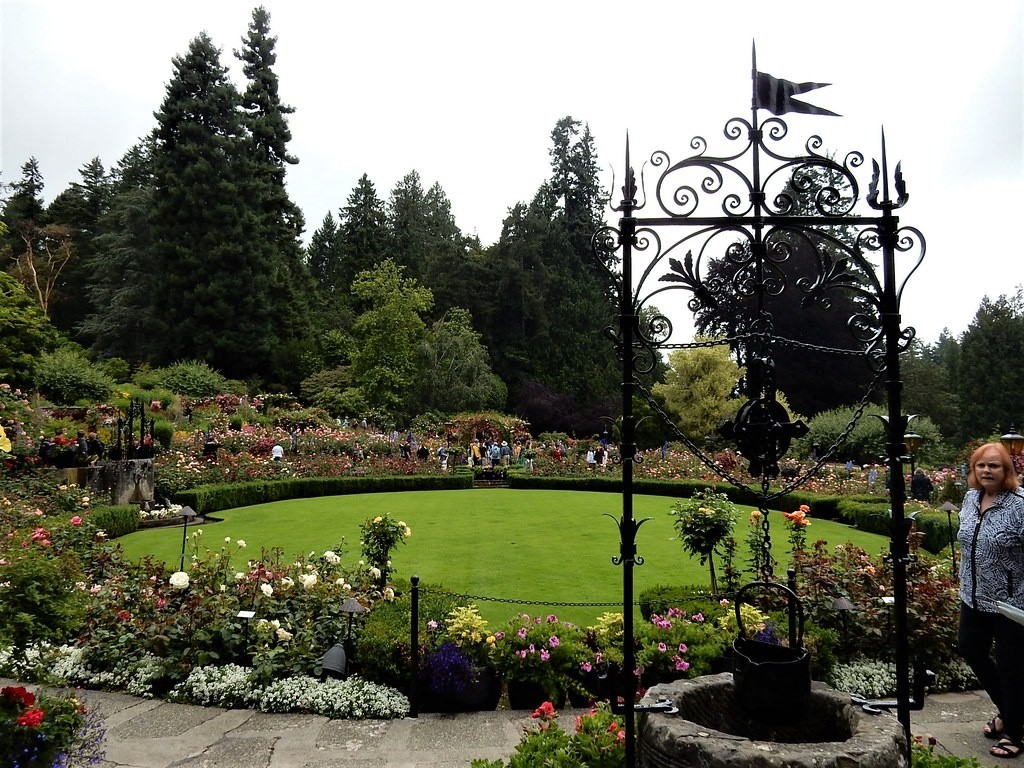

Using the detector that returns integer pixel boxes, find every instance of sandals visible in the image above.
[982,713,1004,739]
[990,734,1024,758]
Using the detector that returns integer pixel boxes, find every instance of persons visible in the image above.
[40,426,105,466]
[910,467,934,503]
[398,438,566,469]
[390,429,412,443]
[956,443,1024,758]
[336,415,367,430]
[587,445,608,472]
[272,444,284,461]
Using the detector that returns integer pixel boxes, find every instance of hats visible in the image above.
[501,441,508,446]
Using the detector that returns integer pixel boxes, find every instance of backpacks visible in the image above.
[492,449,498,458]
